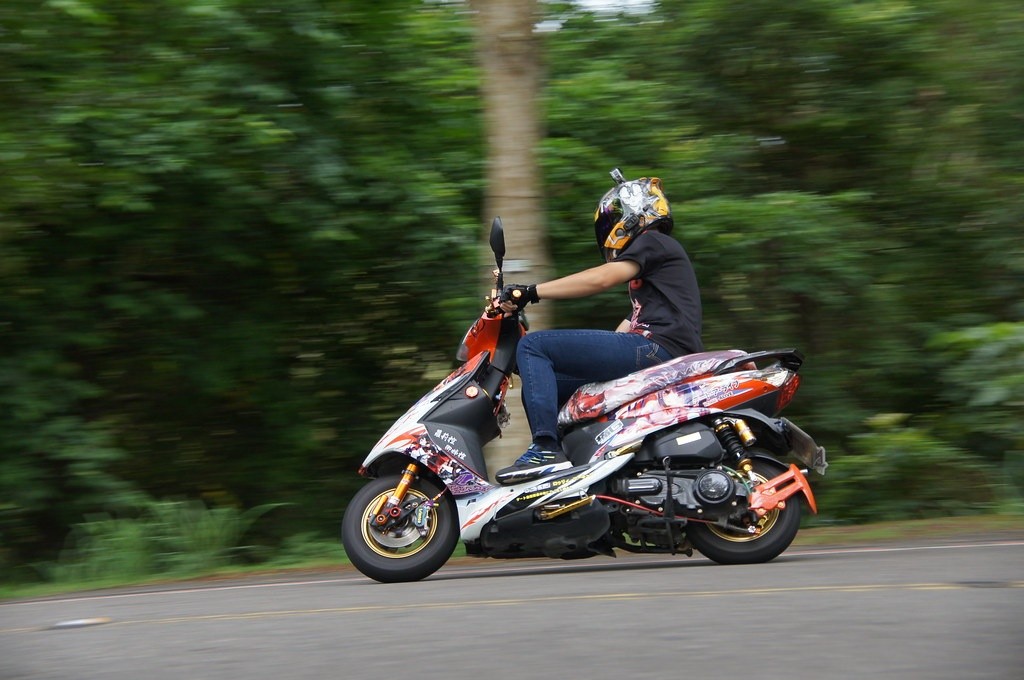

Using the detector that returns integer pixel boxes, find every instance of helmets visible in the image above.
[594,171,672,263]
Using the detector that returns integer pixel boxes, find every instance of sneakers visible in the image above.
[495,441,573,484]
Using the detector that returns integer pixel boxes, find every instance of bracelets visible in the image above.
[530,284,540,304]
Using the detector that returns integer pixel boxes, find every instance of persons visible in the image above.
[496,177,703,483]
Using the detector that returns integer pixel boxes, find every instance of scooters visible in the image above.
[338,215,829,585]
